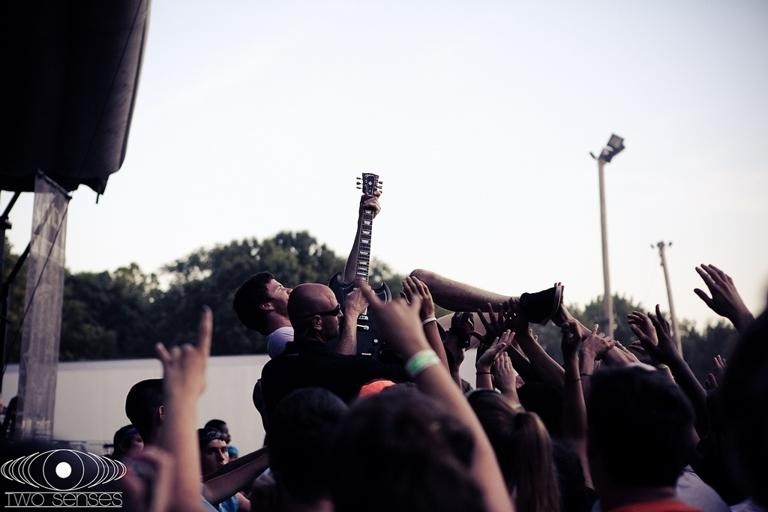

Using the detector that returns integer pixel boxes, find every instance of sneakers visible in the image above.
[517,285,563,326]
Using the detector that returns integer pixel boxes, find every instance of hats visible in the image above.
[358,378,397,400]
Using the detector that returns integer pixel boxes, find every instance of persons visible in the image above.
[111,190,768,512]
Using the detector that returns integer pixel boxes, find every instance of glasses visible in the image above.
[319,302,342,318]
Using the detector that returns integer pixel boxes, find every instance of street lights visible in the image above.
[589,133,626,338]
